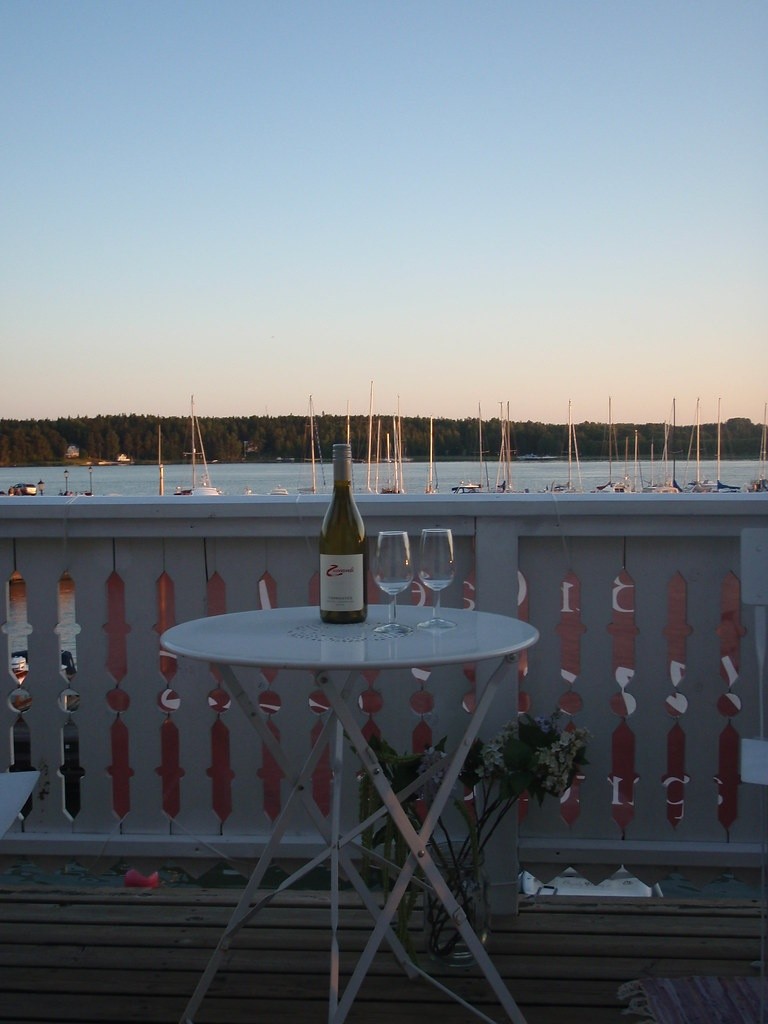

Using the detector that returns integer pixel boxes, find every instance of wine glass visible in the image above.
[417,528,458,629]
[373,530,414,636]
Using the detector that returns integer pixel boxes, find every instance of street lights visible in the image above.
[64,468,69,496]
[38,479,45,496]
[89,466,92,495]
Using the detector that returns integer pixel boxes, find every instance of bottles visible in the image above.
[317,443,370,624]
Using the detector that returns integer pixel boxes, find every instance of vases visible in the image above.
[422,837,493,969]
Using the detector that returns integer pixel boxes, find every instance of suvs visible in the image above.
[8,483,37,496]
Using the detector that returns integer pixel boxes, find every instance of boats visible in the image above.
[242,487,287,495]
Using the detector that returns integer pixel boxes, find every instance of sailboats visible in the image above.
[592,396,740,493]
[538,399,585,494]
[172,394,222,497]
[346,381,444,494]
[742,403,768,493]
[450,402,530,494]
[296,394,332,495]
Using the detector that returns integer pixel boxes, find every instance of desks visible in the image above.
[160,603,542,1024]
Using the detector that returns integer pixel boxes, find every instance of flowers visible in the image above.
[339,706,595,970]
